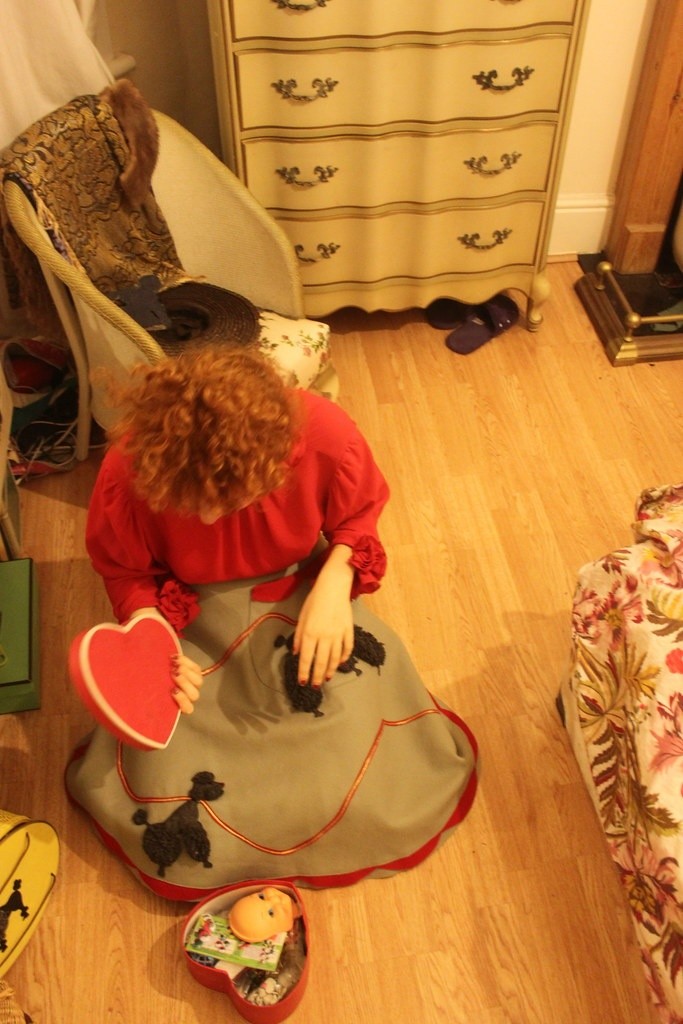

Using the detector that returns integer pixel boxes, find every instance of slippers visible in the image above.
[426,294,519,355]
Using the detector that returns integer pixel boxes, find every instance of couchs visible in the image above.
[554,480,683,1024]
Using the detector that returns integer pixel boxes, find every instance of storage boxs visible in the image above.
[0,557,42,714]
[181,879,312,1024]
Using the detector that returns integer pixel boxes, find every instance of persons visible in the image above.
[63,344,478,902]
[228,888,300,942]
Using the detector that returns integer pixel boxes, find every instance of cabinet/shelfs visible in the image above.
[208,0,592,334]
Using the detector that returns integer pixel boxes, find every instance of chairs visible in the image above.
[3,77,339,459]
